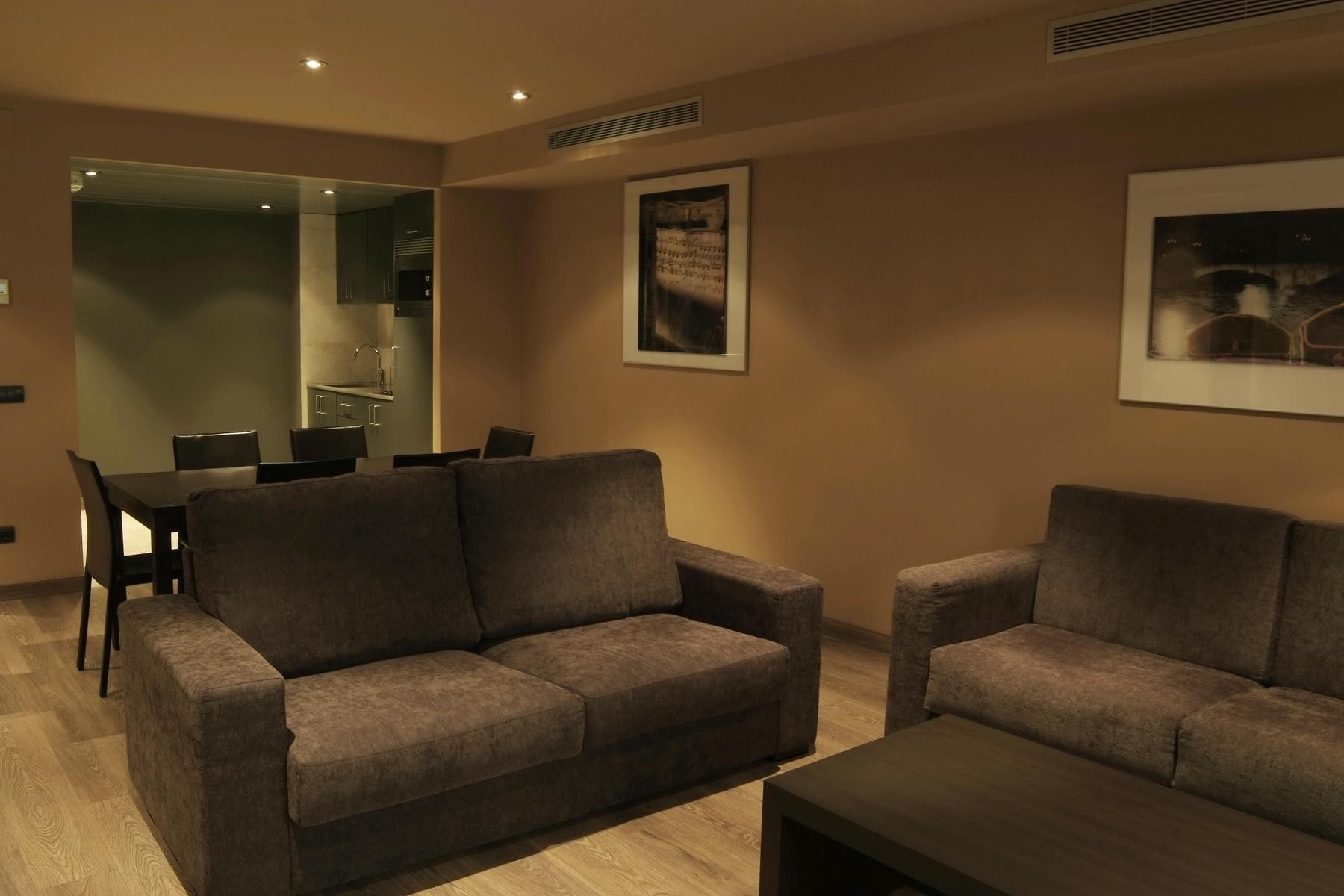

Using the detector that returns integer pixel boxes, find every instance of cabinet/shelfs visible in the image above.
[335,190,434,303]
[306,387,389,459]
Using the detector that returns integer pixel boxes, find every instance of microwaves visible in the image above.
[395,265,432,309]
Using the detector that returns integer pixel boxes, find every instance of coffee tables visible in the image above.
[757,712,1344,895]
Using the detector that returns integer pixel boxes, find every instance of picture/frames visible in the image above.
[1117,156,1344,419]
[622,164,754,374]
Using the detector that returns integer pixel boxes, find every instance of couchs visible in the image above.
[883,483,1343,844]
[116,448,825,896]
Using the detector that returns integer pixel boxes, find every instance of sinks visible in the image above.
[323,384,377,387]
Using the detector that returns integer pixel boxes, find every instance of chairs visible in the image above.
[65,448,183,698]
[172,429,263,471]
[290,423,370,461]
[484,425,535,459]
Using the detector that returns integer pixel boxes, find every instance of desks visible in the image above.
[101,455,395,651]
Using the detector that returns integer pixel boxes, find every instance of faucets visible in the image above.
[353,343,383,386]
